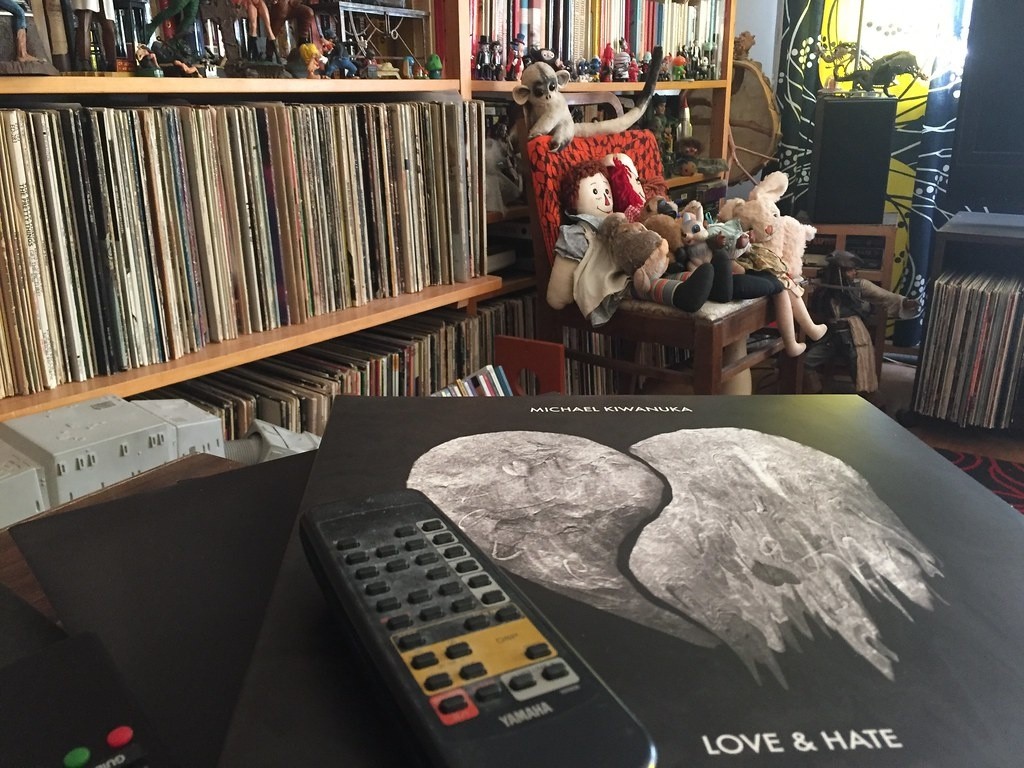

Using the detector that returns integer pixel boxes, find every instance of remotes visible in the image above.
[0,635,178,768]
[299,488,660,768]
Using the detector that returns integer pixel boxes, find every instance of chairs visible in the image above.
[514,92,806,394]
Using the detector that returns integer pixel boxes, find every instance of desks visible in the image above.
[896,212,1024,428]
[803,211,902,383]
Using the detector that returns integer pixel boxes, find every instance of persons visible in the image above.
[796,248,920,394]
[264,0,314,62]
[144,0,198,48]
[70,0,118,71]
[0,0,37,61]
[241,0,282,64]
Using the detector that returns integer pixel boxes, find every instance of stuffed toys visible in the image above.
[546,152,828,356]
[510,46,663,151]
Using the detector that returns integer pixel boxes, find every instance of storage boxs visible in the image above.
[218,395,1024,767]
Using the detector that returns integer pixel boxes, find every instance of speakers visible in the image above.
[808,95,898,225]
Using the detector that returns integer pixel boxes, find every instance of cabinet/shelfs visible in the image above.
[0,0,738,423]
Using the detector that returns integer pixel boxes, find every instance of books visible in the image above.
[126,286,690,442]
[0,99,488,400]
[469,0,725,80]
[909,264,1024,430]
